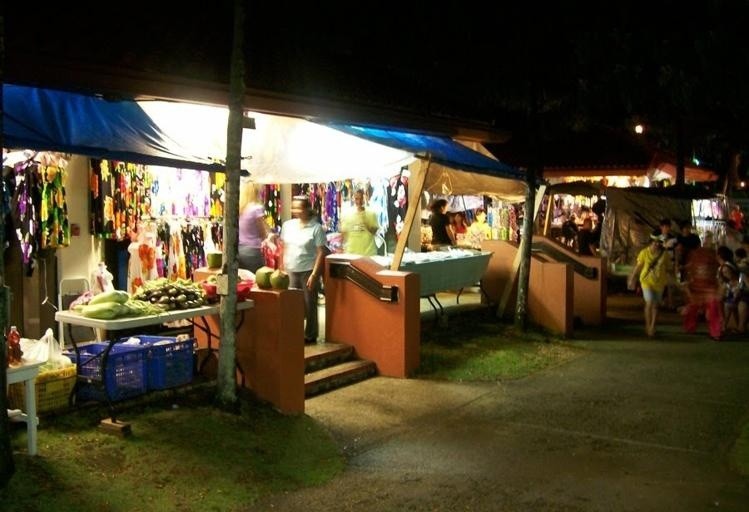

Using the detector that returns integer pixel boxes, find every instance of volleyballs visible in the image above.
[71,290,130,319]
[138,284,205,310]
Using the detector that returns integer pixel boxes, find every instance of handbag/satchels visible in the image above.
[311,272,318,280]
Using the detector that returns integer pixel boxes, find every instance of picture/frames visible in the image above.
[7,336,49,456]
[370,248,491,320]
[53,297,257,438]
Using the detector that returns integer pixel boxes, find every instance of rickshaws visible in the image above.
[207,250,222,267]
[255,266,274,288]
[269,268,289,289]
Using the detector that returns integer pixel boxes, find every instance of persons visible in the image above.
[470,209,491,240]
[428,199,455,250]
[628,219,748,342]
[336,189,379,255]
[238,176,272,269]
[278,194,327,344]
[452,212,467,234]
[607,242,627,266]
[731,204,744,228]
[551,197,604,256]
